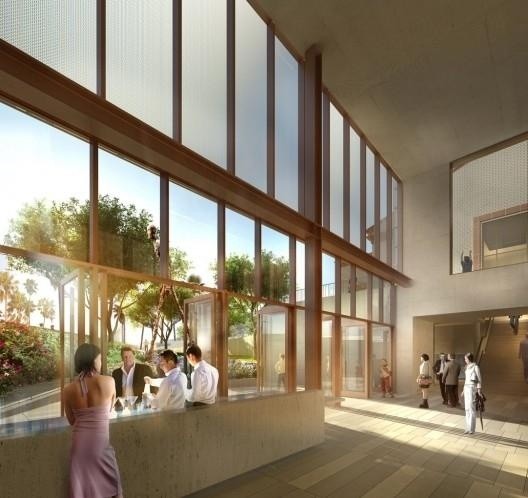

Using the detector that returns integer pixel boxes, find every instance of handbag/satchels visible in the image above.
[417,376,432,387]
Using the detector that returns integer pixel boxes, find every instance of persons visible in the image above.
[183,346,221,407]
[142,350,188,410]
[460,250,472,273]
[509,314,522,334]
[431,352,450,405]
[415,353,431,408]
[111,347,158,404]
[461,352,484,434]
[378,358,394,398]
[61,342,123,497]
[441,351,461,408]
[517,334,528,382]
[275,352,286,392]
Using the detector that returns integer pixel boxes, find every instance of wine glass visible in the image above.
[118,396,138,414]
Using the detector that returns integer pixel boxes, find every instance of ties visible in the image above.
[442,361,446,371]
